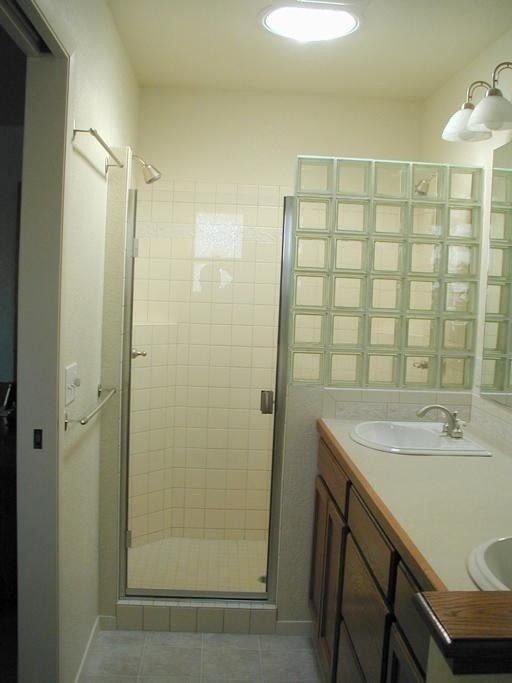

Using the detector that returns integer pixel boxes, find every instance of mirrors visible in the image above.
[480,143,512,408]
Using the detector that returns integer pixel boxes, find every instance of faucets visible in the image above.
[416,404,463,438]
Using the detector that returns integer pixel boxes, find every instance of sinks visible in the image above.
[350,420,492,457]
[468,535,511,592]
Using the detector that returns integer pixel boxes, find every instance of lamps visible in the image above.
[440,61,512,141]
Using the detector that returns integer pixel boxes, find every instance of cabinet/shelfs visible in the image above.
[307,433,437,682]
